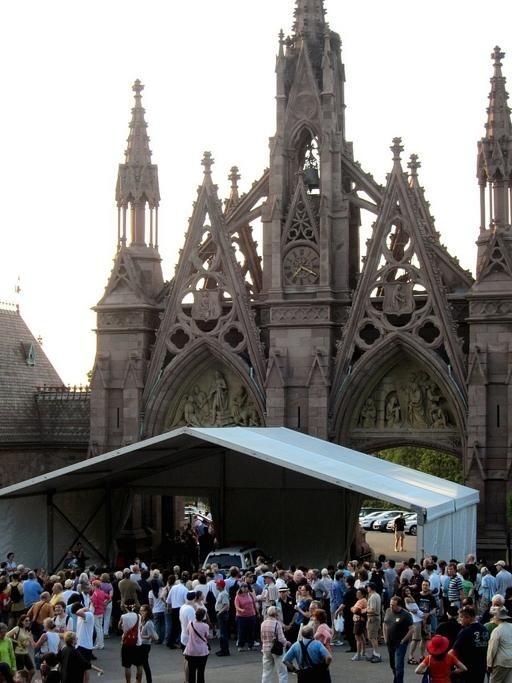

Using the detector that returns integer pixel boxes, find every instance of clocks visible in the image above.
[281,241,324,285]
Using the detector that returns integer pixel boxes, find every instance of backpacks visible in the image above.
[9,582,24,603]
[122,613,140,646]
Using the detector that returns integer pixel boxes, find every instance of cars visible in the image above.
[185,504,213,533]
[359,506,418,536]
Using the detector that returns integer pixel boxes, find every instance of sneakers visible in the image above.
[351,653,361,661]
[329,641,334,645]
[400,548,406,552]
[93,644,105,649]
[371,655,382,663]
[334,640,344,645]
[393,548,399,552]
[213,630,261,652]
[360,654,368,660]
[215,649,231,656]
[366,655,375,661]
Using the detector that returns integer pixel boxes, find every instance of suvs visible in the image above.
[200,546,279,576]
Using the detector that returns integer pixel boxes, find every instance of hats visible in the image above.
[495,608,511,619]
[65,578,76,588]
[425,634,449,655]
[278,584,290,591]
[262,571,273,577]
[187,591,195,600]
[493,560,505,566]
[153,568,160,576]
[214,579,226,586]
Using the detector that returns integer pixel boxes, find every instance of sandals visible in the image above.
[407,657,419,665]
[419,655,425,662]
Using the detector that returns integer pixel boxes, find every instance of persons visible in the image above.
[355,371,451,429]
[181,372,260,426]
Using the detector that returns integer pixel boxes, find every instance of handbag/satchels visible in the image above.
[207,642,212,650]
[151,630,160,641]
[28,620,37,634]
[271,621,284,655]
[12,625,21,652]
[93,625,98,645]
